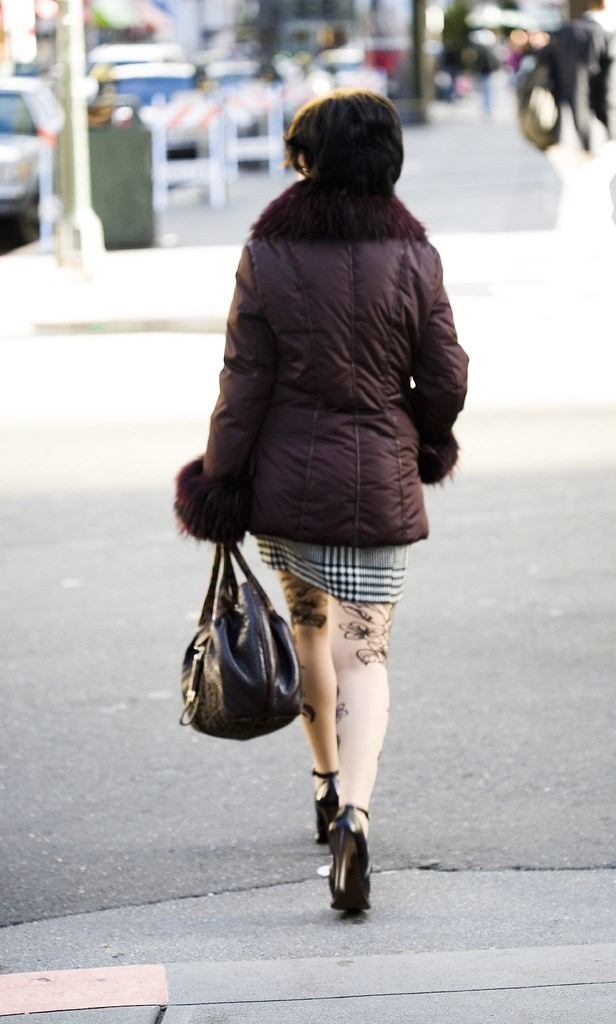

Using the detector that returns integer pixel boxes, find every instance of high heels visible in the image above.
[328,804,372,911]
[312,770,339,844]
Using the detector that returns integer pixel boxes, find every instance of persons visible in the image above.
[432,0,616,162]
[176,90,470,911]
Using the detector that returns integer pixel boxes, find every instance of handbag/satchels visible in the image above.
[179,543,301,741]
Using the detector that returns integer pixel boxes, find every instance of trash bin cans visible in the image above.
[50,95,168,250]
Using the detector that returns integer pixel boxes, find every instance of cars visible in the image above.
[1,40,388,247]
